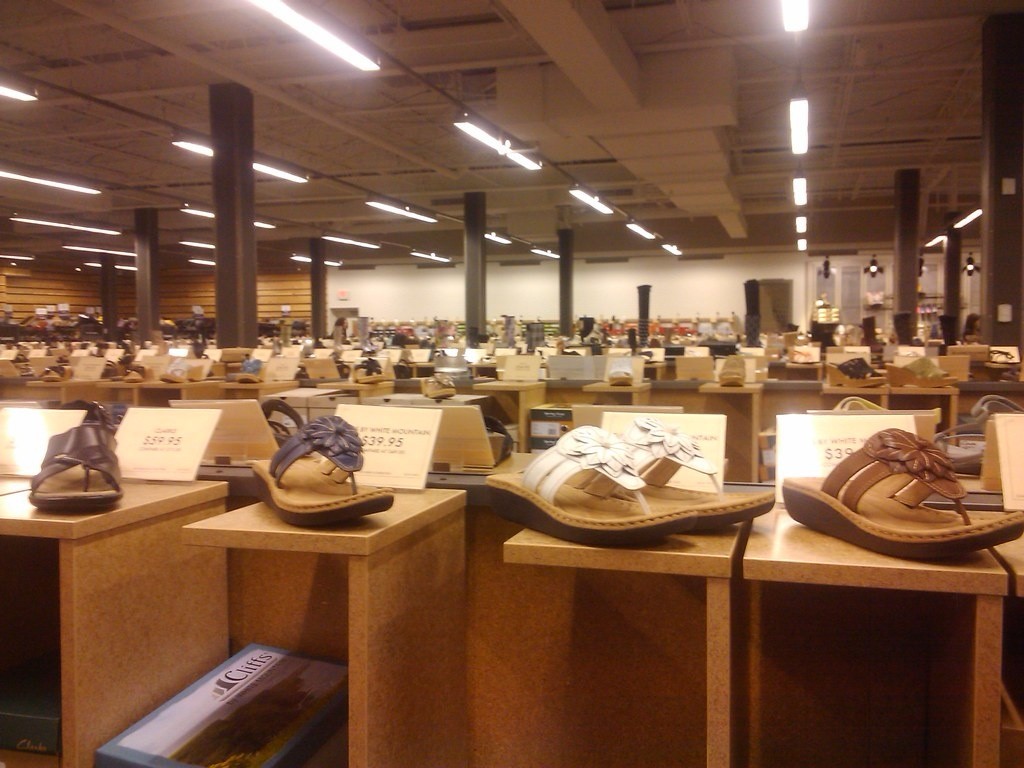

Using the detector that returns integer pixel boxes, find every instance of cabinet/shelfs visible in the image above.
[0,321,1024,768]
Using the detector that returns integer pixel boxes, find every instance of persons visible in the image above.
[963,312,982,342]
[331,317,348,339]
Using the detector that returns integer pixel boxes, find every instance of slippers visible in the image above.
[0,313,1024,560]
